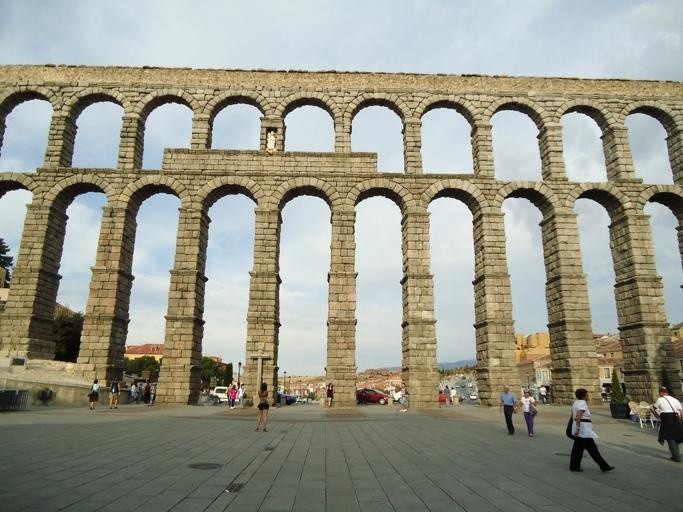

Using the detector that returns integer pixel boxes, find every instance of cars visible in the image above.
[392,390,401,405]
[356,386,389,405]
[439,375,477,402]
[276,391,295,405]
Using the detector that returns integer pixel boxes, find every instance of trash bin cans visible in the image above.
[280,394,286,405]
[402,395,408,405]
[547,393,552,403]
[38,388,53,407]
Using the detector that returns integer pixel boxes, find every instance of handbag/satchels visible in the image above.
[528,404,538,416]
[566,415,576,440]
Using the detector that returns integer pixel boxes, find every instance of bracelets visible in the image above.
[514,408,516,409]
[576,426,580,430]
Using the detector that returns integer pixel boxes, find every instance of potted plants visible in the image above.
[608,367,630,419]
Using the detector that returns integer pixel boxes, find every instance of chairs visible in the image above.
[628,400,662,430]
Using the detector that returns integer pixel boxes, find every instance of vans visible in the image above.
[213,386,239,403]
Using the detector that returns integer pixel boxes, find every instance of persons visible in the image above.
[401,383,409,412]
[327,383,333,406]
[513,388,538,437]
[202,382,245,409]
[254,381,269,432]
[653,387,683,462]
[438,385,457,403]
[498,384,520,435]
[602,384,607,400]
[569,389,616,473]
[363,387,368,404]
[89,379,155,409]
[532,384,546,404]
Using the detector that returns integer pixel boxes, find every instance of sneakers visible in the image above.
[601,466,615,471]
[576,469,583,471]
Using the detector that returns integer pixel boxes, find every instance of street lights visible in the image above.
[288,375,290,394]
[283,371,286,392]
[299,380,301,398]
[236,361,241,383]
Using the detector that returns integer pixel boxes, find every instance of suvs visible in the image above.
[601,382,624,398]
